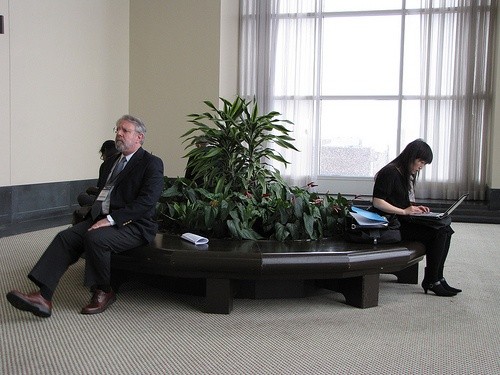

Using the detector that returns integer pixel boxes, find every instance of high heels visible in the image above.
[439,277,462,292]
[421,279,457,297]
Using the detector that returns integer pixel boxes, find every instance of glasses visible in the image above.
[100,154,104,160]
[112,126,141,135]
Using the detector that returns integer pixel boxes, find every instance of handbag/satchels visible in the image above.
[345,214,402,246]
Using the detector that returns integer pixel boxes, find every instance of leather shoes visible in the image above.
[6,288,52,318]
[81,287,117,314]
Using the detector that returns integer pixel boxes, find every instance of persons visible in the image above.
[77,140,119,207]
[185,135,216,188]
[6,115,164,318]
[373,138,462,297]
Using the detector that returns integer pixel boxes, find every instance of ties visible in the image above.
[90,157,126,220]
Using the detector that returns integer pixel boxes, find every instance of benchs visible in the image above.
[79,215,427,316]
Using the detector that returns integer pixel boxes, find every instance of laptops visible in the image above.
[408,194,467,219]
